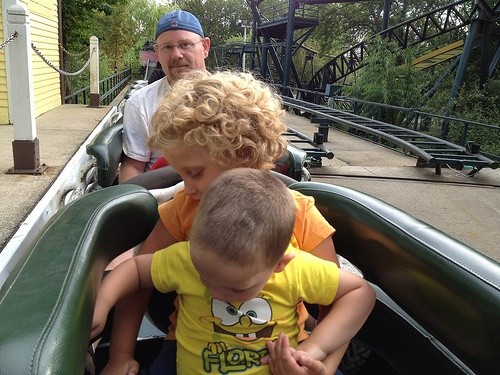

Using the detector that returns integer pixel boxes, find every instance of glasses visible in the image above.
[160,39,202,52]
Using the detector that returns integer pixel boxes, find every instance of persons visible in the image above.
[118,10,210,185]
[90,167,376,375]
[98,67,352,375]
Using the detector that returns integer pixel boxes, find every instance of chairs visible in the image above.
[87,120,307,186]
[0,183,500,375]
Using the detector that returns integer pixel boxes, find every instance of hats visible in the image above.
[156,9,205,37]
